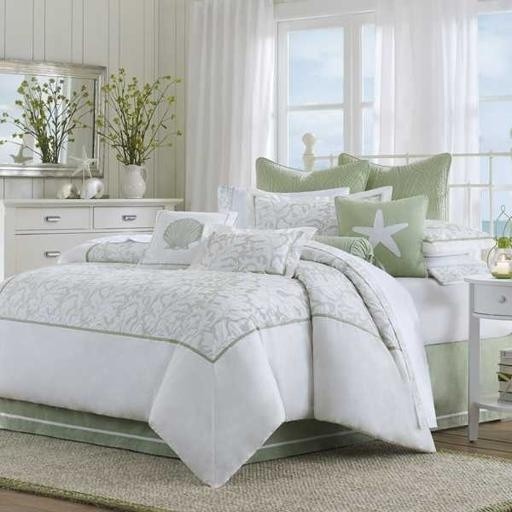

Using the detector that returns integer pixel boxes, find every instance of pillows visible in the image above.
[254,185,394,234]
[339,152,452,219]
[137,208,240,266]
[335,194,428,280]
[185,222,317,279]
[218,184,350,229]
[256,156,370,193]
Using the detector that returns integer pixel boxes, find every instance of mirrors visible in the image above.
[1,57,109,176]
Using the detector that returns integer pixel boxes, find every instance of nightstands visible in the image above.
[461,273,512,442]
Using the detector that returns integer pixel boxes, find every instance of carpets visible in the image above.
[1,426,512,512]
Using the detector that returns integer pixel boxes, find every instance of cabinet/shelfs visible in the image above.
[2,198,184,278]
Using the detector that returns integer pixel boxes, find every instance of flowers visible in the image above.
[1,77,91,164]
[91,67,184,165]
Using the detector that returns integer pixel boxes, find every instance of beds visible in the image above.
[1,129,512,486]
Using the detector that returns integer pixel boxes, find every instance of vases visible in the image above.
[118,165,145,198]
